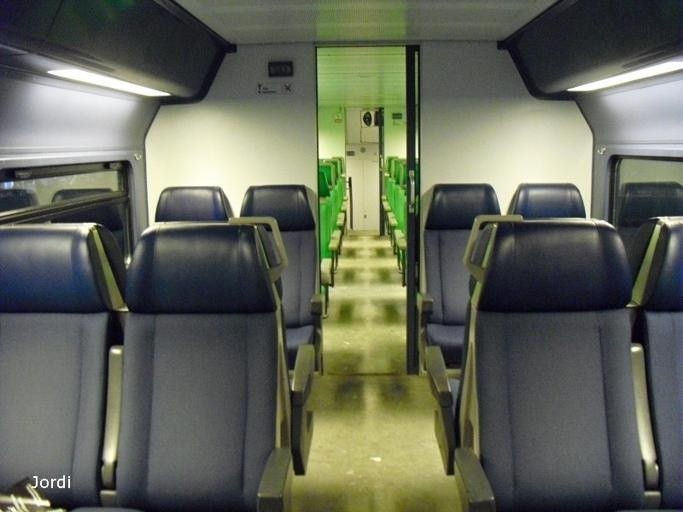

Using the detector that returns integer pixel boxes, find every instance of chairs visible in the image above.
[0,183,327,512]
[381,157,409,287]
[410,181,682,512]
[319,156,348,287]
